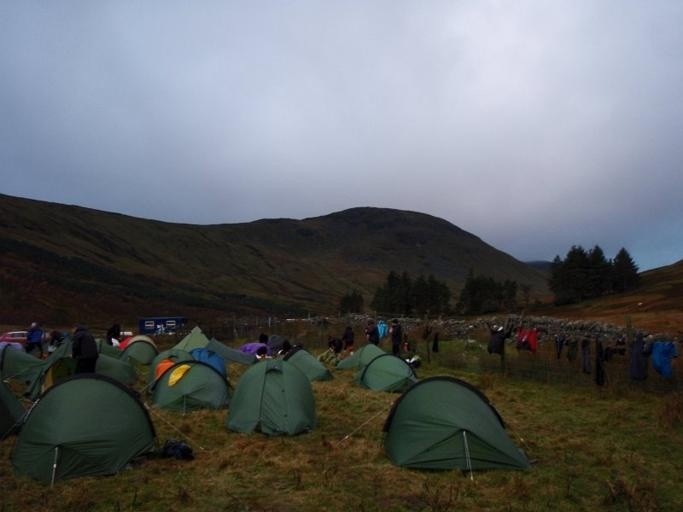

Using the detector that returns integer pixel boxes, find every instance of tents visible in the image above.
[334,344,386,370]
[355,353,419,394]
[0,325,291,441]
[10,373,161,487]
[225,358,315,435]
[285,348,332,379]
[378,374,530,475]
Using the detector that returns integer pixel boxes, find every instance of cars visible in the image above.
[0,331,28,347]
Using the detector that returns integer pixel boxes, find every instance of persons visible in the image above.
[319,315,412,361]
[23,320,43,359]
